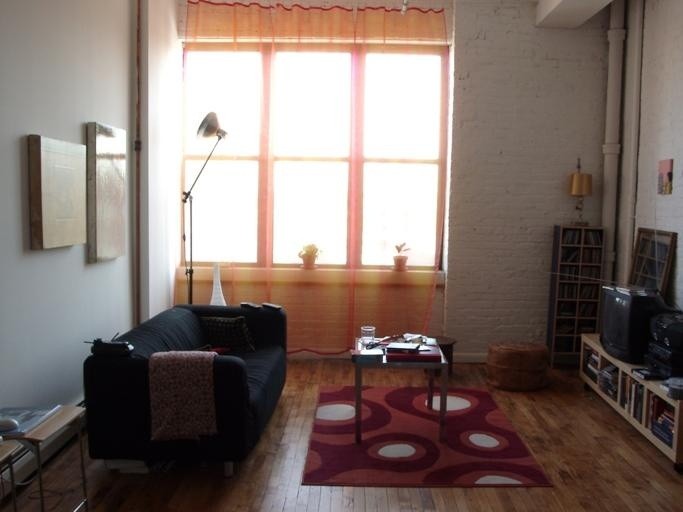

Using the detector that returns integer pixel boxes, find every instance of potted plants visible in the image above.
[391,243,411,272]
[298,244,320,270]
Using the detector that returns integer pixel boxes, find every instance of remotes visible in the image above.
[241,301,261,308]
[262,303,282,310]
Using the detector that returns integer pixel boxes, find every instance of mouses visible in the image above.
[0,418,18,431]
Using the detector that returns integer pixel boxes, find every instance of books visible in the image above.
[385,344,441,363]
[0,402,60,437]
[351,349,383,362]
[586,349,674,448]
[386,342,420,352]
[554,227,602,352]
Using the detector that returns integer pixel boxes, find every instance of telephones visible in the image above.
[84,338,135,355]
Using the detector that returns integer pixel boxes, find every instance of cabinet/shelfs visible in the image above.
[546,224,607,369]
[632,237,670,292]
[578,333,683,475]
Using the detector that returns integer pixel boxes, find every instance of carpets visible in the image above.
[302,383,556,487]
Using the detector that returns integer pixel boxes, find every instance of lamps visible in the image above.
[182,111,228,305]
[569,157,592,227]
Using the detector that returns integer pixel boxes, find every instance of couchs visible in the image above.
[83,304,287,478]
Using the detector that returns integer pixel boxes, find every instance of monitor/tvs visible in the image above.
[599,286,661,363]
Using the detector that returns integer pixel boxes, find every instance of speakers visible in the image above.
[649,313,683,376]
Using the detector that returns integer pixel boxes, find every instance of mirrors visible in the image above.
[629,227,677,297]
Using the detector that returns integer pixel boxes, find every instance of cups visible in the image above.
[361,326,375,345]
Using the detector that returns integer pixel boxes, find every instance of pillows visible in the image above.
[199,315,255,353]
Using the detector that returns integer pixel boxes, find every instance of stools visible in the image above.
[487,340,549,392]
[424,336,457,377]
[0,405,88,512]
